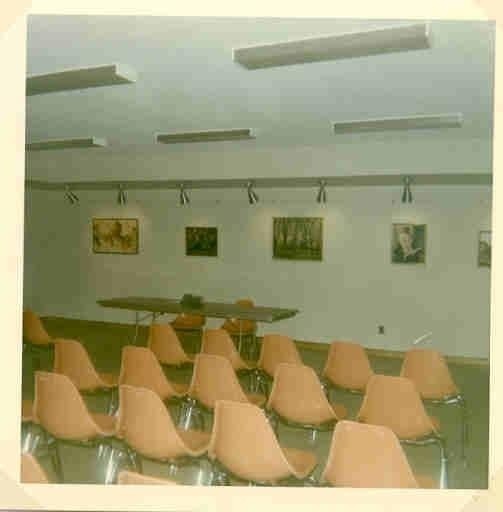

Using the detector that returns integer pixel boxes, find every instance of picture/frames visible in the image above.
[92,217,140,255]
[184,225,218,257]
[272,216,324,261]
[477,229,490,266]
[390,223,427,264]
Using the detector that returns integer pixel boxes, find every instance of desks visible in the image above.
[97,296,299,359]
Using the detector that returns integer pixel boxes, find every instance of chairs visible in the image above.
[221,298,258,342]
[355,374,449,489]
[399,346,466,409]
[172,314,208,336]
[118,469,174,484]
[256,334,304,377]
[32,368,117,482]
[183,352,268,431]
[200,328,257,395]
[264,361,348,432]
[119,383,212,472]
[323,341,374,395]
[20,399,34,423]
[118,344,189,404]
[21,450,50,483]
[148,323,190,370]
[318,419,441,489]
[53,338,120,416]
[207,397,318,487]
[23,309,62,347]
